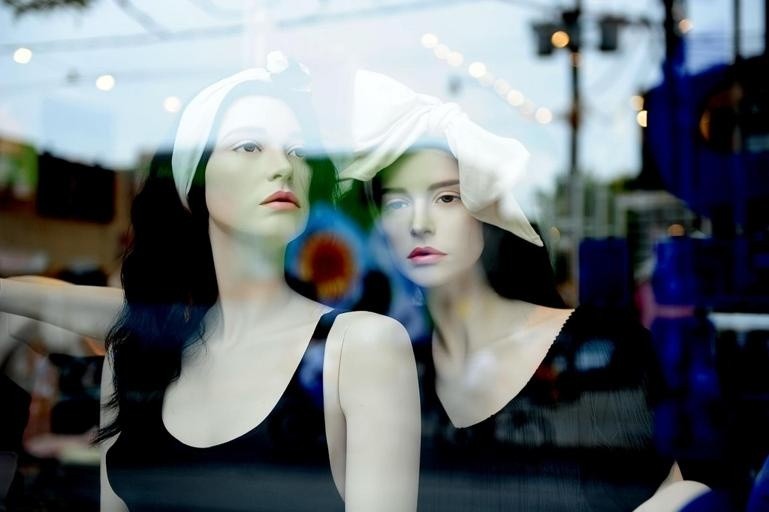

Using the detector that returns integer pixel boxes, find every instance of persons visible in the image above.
[321,73,706,511]
[639,224,713,453]
[100,54,421,512]
[1,274,125,512]
